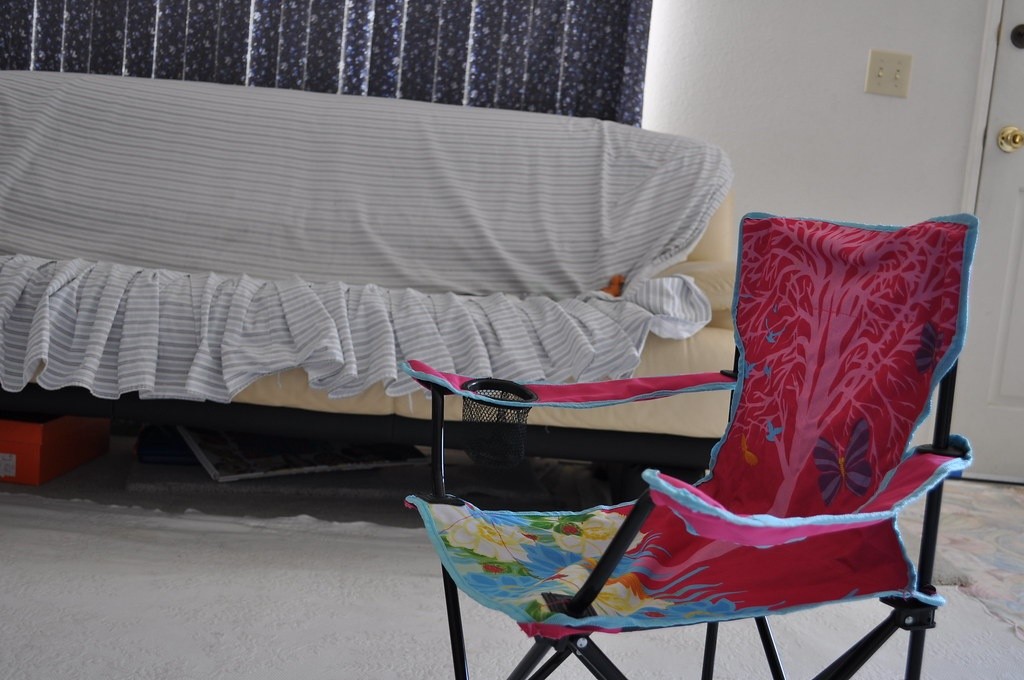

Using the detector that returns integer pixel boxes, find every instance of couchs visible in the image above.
[1,70,740,472]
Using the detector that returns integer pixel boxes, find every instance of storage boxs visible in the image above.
[0,414,109,487]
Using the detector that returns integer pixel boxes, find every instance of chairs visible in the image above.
[401,212,980,680]
